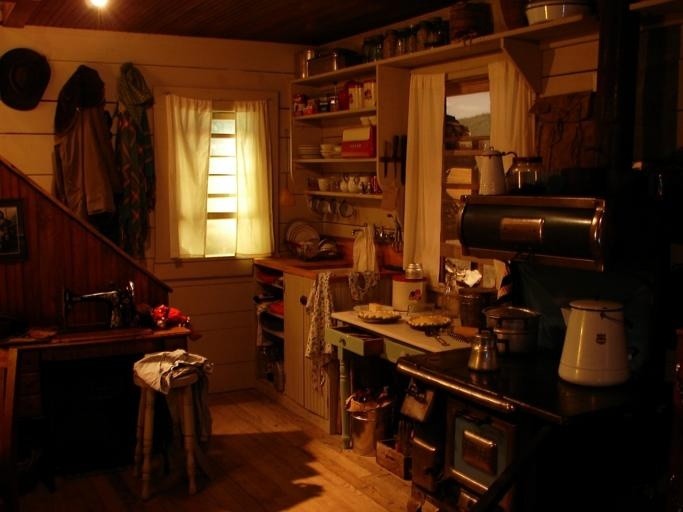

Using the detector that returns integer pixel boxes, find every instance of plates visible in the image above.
[353,303,452,328]
[284,220,320,254]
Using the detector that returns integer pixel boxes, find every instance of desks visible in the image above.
[2,318,192,512]
[396,338,620,511]
[323,300,481,448]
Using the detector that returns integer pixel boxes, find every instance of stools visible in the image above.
[132,354,201,500]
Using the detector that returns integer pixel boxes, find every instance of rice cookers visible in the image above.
[392,275,426,311]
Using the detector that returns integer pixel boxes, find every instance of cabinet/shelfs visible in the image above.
[286,1,663,201]
[253,249,403,438]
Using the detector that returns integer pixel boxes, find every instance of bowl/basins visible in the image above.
[318,238,339,256]
[524,4,588,26]
[298,143,334,158]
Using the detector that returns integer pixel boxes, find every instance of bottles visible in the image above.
[293,93,304,115]
[406,263,425,279]
[327,96,339,112]
[505,156,548,195]
[361,15,447,63]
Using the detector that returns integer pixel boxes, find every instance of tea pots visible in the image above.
[473,147,517,195]
[558,296,629,387]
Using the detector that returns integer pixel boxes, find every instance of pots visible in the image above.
[478,304,545,368]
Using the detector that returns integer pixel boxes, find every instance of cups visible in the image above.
[469,331,496,371]
[317,170,382,194]
[483,263,495,288]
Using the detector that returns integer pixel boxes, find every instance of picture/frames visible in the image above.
[0,198,29,261]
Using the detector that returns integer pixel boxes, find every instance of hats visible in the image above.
[1,47,51,111]
